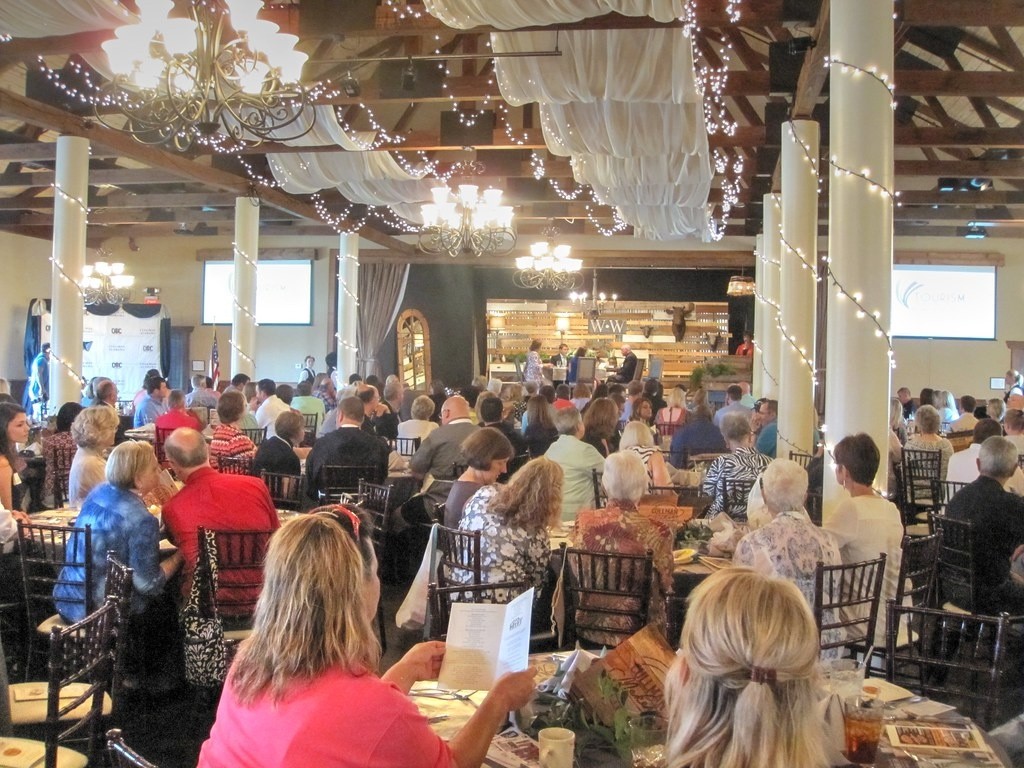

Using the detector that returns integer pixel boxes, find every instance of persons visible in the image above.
[0,331,1024,768]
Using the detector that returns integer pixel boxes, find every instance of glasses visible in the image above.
[369,395,381,401]
[113,388,118,396]
[759,411,771,415]
[561,348,570,350]
[437,409,449,419]
[162,456,174,464]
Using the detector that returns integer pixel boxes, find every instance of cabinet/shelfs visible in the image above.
[485,299,732,396]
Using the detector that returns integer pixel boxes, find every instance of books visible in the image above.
[886,725,1001,768]
[436,587,535,690]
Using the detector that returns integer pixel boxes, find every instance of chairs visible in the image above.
[1,404,1024,768]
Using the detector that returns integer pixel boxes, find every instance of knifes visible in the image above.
[902,709,971,725]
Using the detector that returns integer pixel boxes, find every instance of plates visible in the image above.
[672,548,695,564]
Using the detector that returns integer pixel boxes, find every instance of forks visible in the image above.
[407,689,478,700]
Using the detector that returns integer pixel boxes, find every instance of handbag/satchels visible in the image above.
[395,522,450,641]
[551,546,567,648]
[179,527,229,688]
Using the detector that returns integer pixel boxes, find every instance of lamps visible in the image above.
[93,0,316,153]
[570,269,618,321]
[402,55,417,90]
[83,247,134,303]
[727,266,756,296]
[343,64,361,99]
[512,216,584,292]
[417,160,518,258]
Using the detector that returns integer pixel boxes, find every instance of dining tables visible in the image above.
[124,426,214,447]
[407,650,1012,768]
[22,507,304,558]
[299,458,414,485]
[545,521,853,650]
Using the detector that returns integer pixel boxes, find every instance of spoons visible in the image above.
[903,749,960,761]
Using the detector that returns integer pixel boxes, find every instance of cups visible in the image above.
[538,727,576,768]
[628,715,670,768]
[830,658,884,768]
[76,499,86,512]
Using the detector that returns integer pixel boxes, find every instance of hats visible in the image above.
[751,398,769,410]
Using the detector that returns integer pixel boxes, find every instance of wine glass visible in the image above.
[556,360,567,367]
